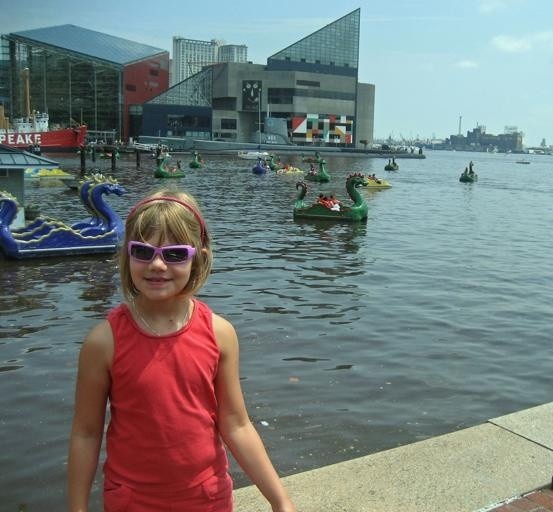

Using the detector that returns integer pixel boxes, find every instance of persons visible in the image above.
[470,160,473,173]
[346,172,378,180]
[151,148,204,172]
[69,191,298,511]
[257,153,317,176]
[318,194,341,209]
[393,155,396,164]
[88,137,124,156]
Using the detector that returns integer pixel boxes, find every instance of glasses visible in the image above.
[126,240,196,266]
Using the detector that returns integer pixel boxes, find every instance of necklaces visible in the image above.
[130,296,192,336]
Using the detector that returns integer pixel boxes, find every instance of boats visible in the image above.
[383,156,399,171]
[293,170,368,222]
[154,153,185,179]
[188,154,208,168]
[0,111,86,151]
[97,148,120,160]
[0,171,125,260]
[366,178,392,188]
[459,161,478,182]
[252,151,330,183]
[237,151,270,161]
